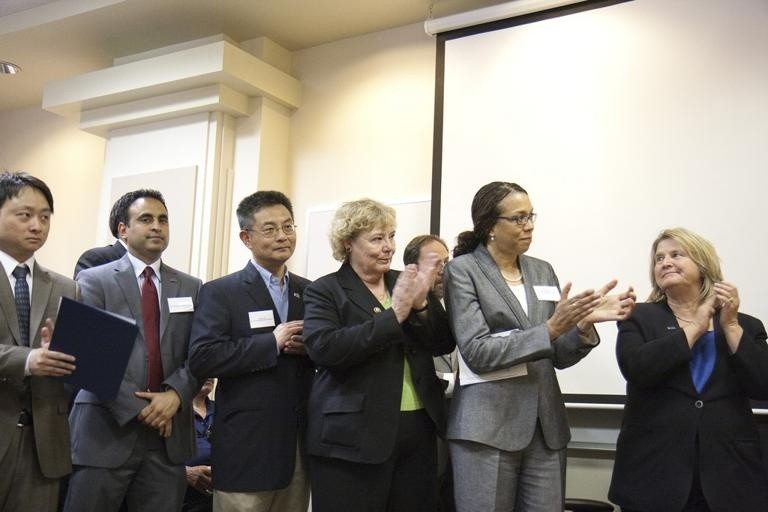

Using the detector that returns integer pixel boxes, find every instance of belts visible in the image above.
[17,411,31,428]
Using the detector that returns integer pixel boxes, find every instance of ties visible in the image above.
[141,267,163,392]
[11,266,30,348]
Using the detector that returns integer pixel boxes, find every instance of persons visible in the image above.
[302,197,456,511]
[607,226,767,512]
[441,179,639,512]
[73,191,134,277]
[189,188,314,510]
[61,189,207,512]
[0,170,81,512]
[403,234,457,512]
[182,377,216,512]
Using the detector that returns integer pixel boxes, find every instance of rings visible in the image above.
[571,302,578,310]
[728,294,733,300]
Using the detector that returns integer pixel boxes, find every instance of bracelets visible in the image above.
[410,305,428,313]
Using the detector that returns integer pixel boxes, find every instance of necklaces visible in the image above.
[673,313,693,324]
[500,271,522,283]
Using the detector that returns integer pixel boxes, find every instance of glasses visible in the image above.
[497,212,537,226]
[247,223,298,238]
